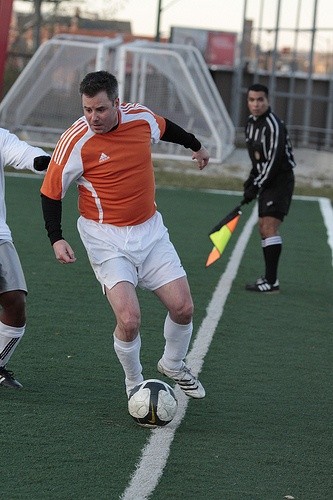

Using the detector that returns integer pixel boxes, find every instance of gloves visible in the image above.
[244,177,254,190]
[244,185,259,204]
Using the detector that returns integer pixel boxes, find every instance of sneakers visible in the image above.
[157,358,206,399]
[0,362,23,389]
[246,277,279,293]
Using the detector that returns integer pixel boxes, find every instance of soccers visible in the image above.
[127,378,179,428]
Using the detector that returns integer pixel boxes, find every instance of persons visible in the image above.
[243,84,296,292]
[0,128,52,391]
[40,71,211,399]
